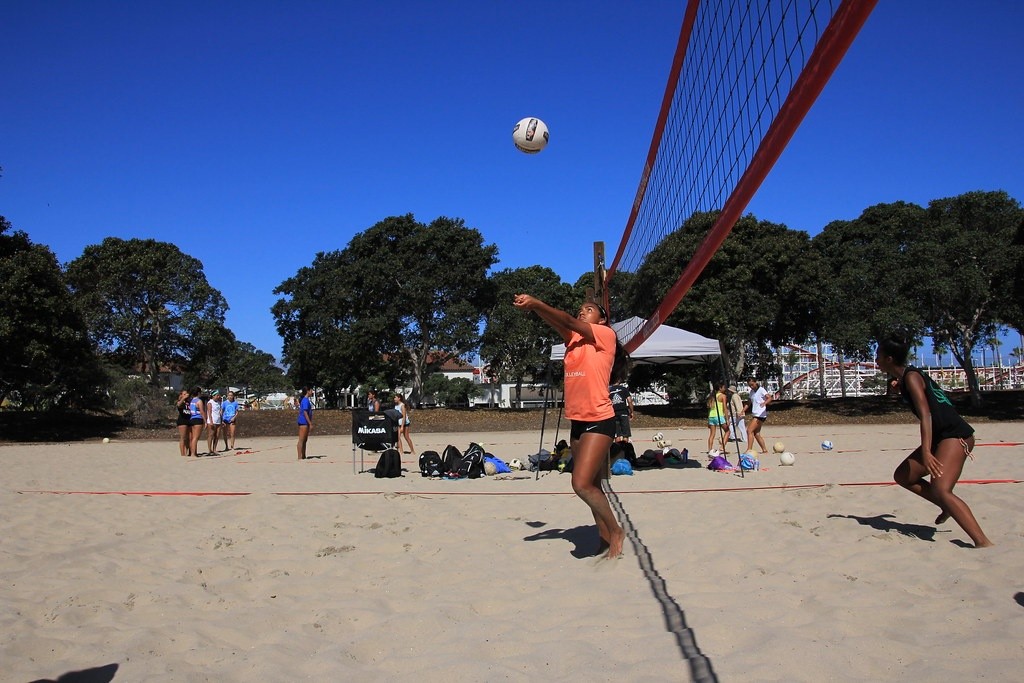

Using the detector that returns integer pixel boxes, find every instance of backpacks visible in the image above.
[419,442,485,479]
[375,450,401,478]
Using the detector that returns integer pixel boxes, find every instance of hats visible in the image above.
[728,385,738,393]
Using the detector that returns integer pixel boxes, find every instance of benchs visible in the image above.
[513,401,564,408]
[474,403,499,408]
[447,403,467,408]
[417,404,437,409]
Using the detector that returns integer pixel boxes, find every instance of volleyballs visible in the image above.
[780,452,795,466]
[821,440,833,450]
[483,461,496,475]
[746,450,758,458]
[512,116,550,155]
[652,431,664,441]
[657,440,665,448]
[708,448,720,460]
[103,437,110,443]
[509,459,523,470]
[773,442,784,453]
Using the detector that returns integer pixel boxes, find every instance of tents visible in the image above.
[536,315,745,480]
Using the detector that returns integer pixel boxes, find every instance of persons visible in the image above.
[394,393,416,455]
[220,391,239,451]
[608,373,633,442]
[368,389,381,453]
[514,293,633,560]
[177,389,191,456]
[707,381,730,454]
[728,385,748,442]
[206,390,221,455]
[297,385,314,461]
[739,376,772,454]
[188,385,208,456]
[875,328,995,548]
